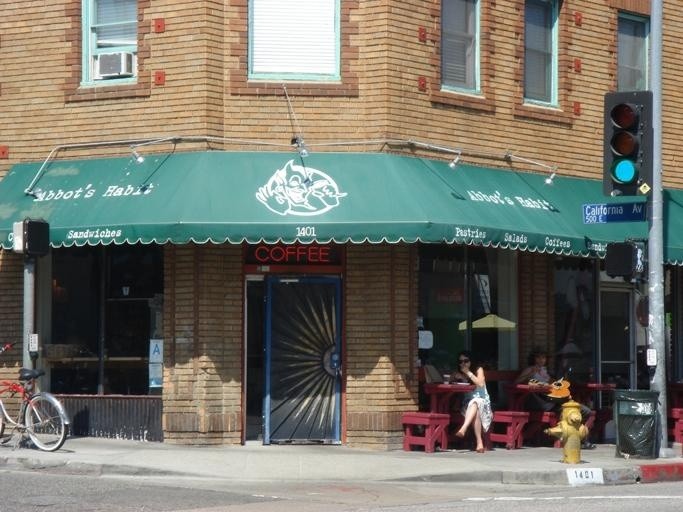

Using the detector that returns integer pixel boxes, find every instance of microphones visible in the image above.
[402,413,450,453]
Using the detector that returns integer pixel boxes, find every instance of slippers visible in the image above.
[423,365,443,384]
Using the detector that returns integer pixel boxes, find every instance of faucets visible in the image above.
[476,447,484,453]
[456,432,464,439]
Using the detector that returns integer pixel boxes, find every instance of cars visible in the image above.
[443,374,450,385]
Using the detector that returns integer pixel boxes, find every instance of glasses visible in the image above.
[506,152,556,186]
[292,137,308,158]
[129,137,180,165]
[409,139,462,171]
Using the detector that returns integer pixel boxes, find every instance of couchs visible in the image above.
[600,89,655,200]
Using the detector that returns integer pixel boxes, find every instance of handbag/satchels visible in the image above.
[467,371,473,378]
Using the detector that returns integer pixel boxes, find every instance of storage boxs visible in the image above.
[489,408,683,449]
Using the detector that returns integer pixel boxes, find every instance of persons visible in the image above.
[448,351,493,453]
[512,348,597,449]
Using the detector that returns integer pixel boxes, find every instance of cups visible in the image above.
[458,359,469,363]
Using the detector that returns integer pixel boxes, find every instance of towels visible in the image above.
[612,390,660,459]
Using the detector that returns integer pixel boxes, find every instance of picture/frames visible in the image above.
[423,382,475,443]
[574,382,617,403]
[669,382,683,407]
[504,384,549,411]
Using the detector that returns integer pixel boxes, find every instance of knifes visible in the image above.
[98,52,134,78]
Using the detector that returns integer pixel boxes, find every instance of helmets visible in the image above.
[457,382,470,385]
